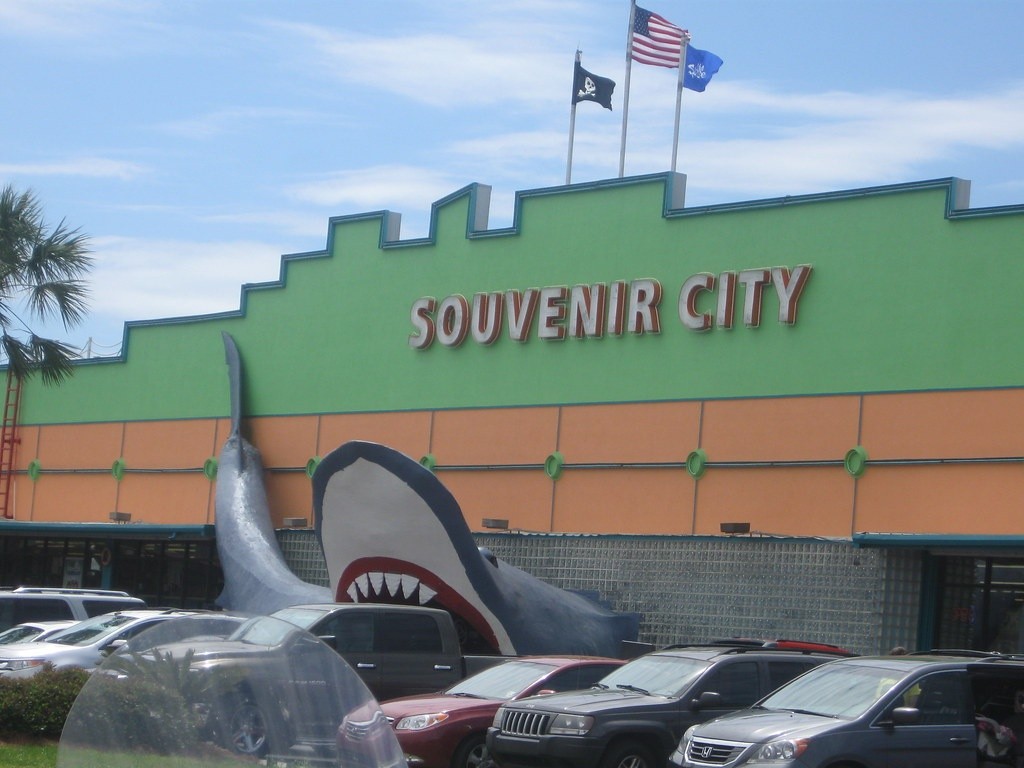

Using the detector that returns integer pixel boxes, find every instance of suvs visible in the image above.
[0,585,146,634]
[486,640,865,768]
[0,607,253,683]
[666,649,1024,768]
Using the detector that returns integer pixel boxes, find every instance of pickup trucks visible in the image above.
[129,602,519,760]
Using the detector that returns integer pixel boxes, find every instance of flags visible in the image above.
[682,44,723,93]
[571,61,615,110]
[626,4,690,69]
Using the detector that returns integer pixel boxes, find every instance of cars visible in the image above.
[335,654,628,768]
[0,620,83,645]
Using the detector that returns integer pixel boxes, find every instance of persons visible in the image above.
[879,647,921,707]
